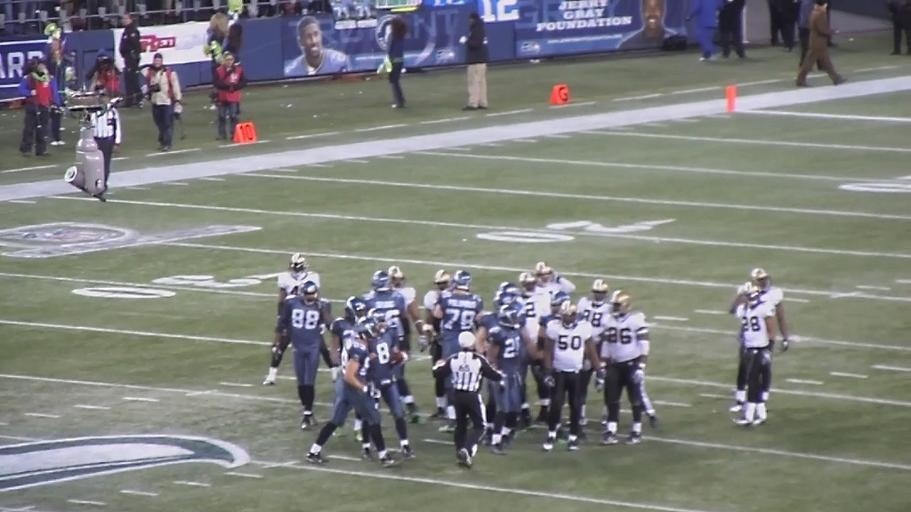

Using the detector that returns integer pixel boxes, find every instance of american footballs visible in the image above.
[389,352,403,369]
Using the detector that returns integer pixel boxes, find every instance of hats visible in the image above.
[459,331,477,349]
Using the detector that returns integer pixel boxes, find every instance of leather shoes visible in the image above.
[463,105,478,110]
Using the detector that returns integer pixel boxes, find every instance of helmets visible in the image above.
[388,265,405,289]
[591,279,609,306]
[347,295,368,322]
[289,253,309,271]
[371,270,391,294]
[533,260,555,282]
[453,269,471,289]
[610,289,633,317]
[368,308,387,334]
[497,304,526,331]
[298,282,318,306]
[354,316,379,341]
[519,272,536,296]
[497,281,522,296]
[750,268,770,292]
[492,293,523,314]
[742,282,760,306]
[559,299,579,326]
[550,290,570,313]
[433,270,451,288]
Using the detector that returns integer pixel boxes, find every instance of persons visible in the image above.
[284,17,351,77]
[389,265,428,349]
[19,36,70,157]
[798,0,822,69]
[478,262,660,457]
[330,296,370,441]
[794,1,847,87]
[729,266,790,413]
[89,88,122,190]
[770,0,800,51]
[423,269,452,419]
[687,0,723,62]
[889,2,910,54]
[358,271,420,423]
[274,281,332,429]
[213,50,249,140]
[432,332,505,467]
[147,52,183,150]
[119,12,144,108]
[263,252,337,386]
[385,17,409,110]
[118,0,332,27]
[729,282,776,426]
[459,12,491,112]
[1,0,118,29]
[433,269,484,433]
[305,316,397,467]
[718,0,747,58]
[617,0,678,51]
[361,307,415,462]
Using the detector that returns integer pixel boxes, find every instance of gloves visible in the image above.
[762,350,774,364]
[632,367,644,384]
[272,343,283,361]
[391,346,401,363]
[781,339,792,351]
[364,384,377,397]
[593,377,605,393]
[544,375,556,387]
[416,336,429,349]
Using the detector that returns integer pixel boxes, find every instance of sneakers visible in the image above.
[536,407,546,425]
[622,432,642,445]
[407,415,420,424]
[731,417,753,425]
[361,447,374,461]
[479,426,491,447]
[579,417,588,427]
[379,452,400,467]
[457,447,472,467]
[353,429,364,442]
[470,444,478,459]
[602,406,609,425]
[333,427,346,438]
[600,431,619,444]
[751,418,766,428]
[522,408,533,427]
[650,416,657,429]
[567,439,580,451]
[490,443,506,454]
[542,436,555,452]
[430,408,447,419]
[301,412,317,430]
[262,376,275,384]
[730,401,743,412]
[401,444,416,462]
[439,425,456,432]
[503,429,515,442]
[305,452,322,463]
